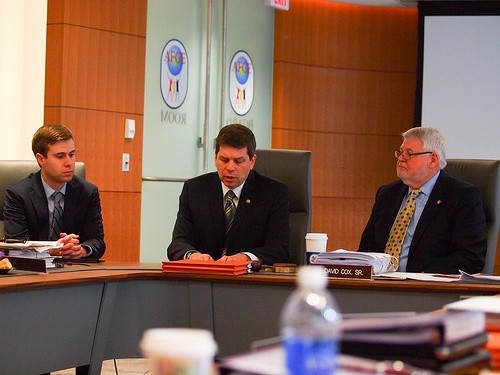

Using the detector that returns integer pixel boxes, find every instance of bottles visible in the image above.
[280,266,341,375]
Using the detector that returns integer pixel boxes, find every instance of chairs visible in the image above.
[254,149,312,264]
[443,159,500,275]
[0,159,86,239]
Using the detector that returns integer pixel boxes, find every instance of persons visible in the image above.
[3,123,106,262]
[357,127,488,275]
[166,123,291,268]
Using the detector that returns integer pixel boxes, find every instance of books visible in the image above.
[444,296,500,327]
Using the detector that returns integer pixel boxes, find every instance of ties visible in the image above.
[224,190,236,237]
[51,191,64,241]
[384,189,421,272]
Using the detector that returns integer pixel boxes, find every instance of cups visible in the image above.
[305,232,328,265]
[140,327,217,375]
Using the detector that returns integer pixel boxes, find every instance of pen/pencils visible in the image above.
[431,275,460,279]
[4,238,26,243]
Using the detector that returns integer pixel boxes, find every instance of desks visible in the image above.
[0,260,500,375]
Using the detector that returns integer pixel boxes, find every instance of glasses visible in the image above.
[393,149,433,160]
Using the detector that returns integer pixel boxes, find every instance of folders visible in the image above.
[344,309,486,345]
